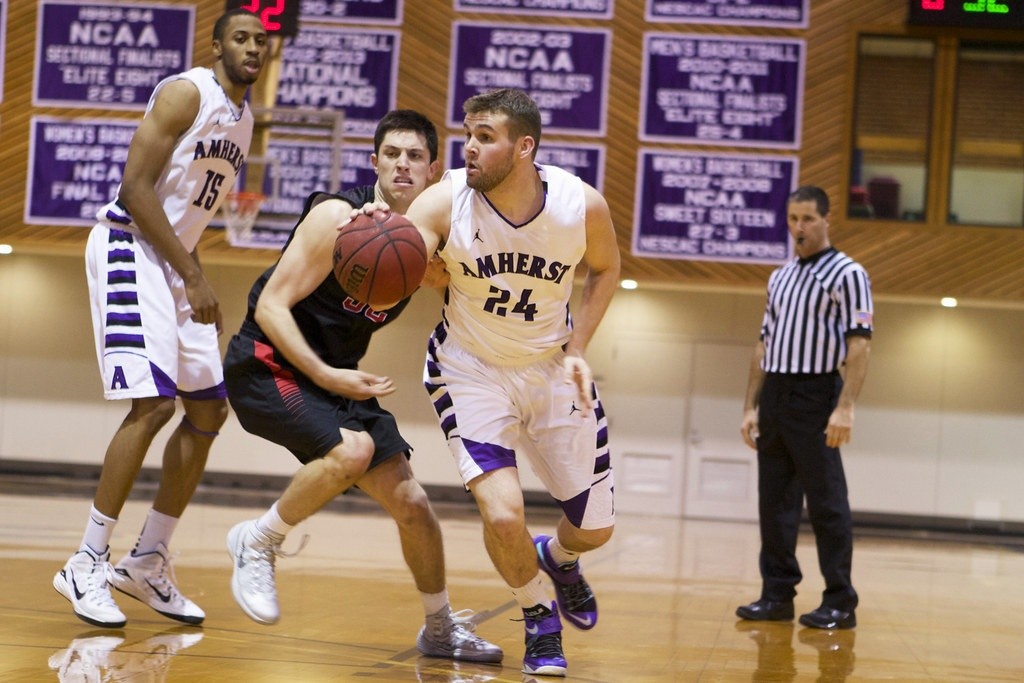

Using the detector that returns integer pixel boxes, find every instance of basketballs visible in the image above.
[332,213,428,305]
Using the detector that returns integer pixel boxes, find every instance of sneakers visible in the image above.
[108,625,203,683]
[533,535,598,630]
[53,544,127,628]
[227,520,310,625]
[509,600,568,676]
[416,603,504,662]
[48,630,125,683]
[107,542,206,625]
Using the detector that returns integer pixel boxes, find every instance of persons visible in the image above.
[51,7,270,625]
[335,85,622,676]
[735,186,874,633]
[223,109,504,666]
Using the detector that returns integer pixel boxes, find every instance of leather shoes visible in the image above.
[798,627,856,652]
[736,599,794,621]
[735,619,794,646]
[799,603,856,629]
